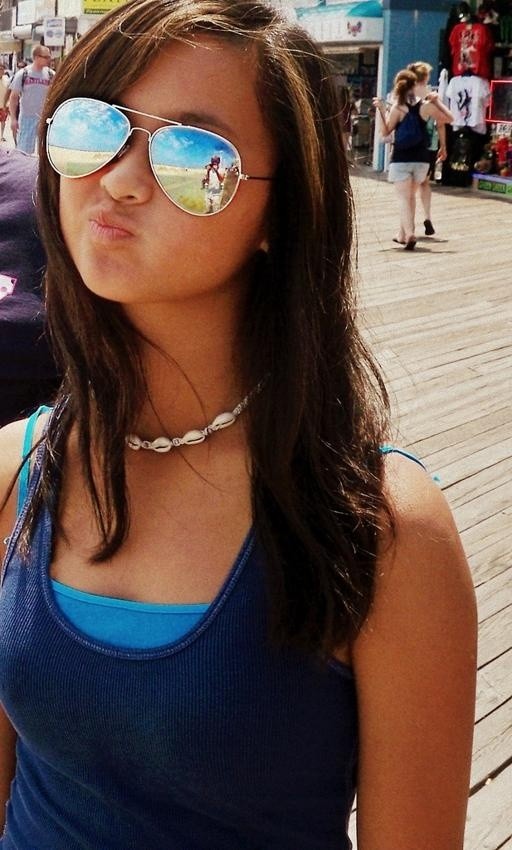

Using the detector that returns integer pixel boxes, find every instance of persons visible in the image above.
[370,62,453,249]
[0,45,56,154]
[1,1,477,850]
[439,15,486,188]
[201,155,225,213]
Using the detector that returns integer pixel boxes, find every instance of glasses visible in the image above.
[38,55,51,59]
[45,97,274,217]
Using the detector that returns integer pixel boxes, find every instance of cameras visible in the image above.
[210,164,216,168]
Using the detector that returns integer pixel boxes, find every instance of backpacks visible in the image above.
[378,97,426,152]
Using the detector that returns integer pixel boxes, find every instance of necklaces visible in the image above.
[123,371,271,453]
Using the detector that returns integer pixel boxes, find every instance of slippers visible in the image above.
[392,235,416,250]
[424,220,435,235]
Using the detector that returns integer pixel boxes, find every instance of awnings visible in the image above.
[298,0,385,45]
